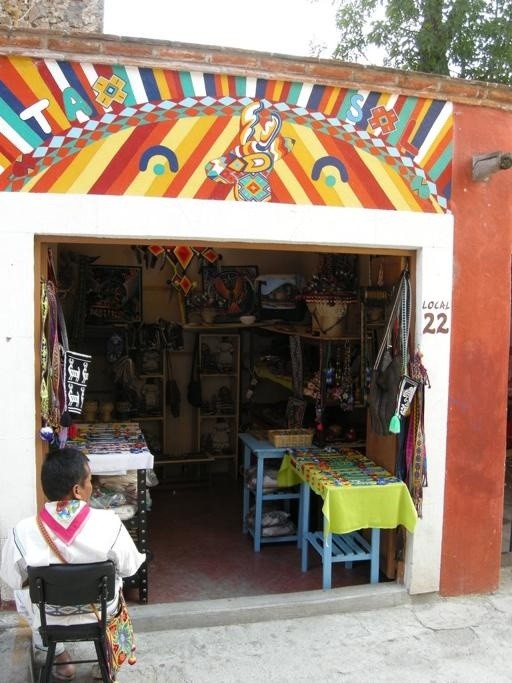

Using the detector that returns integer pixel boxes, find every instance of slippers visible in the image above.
[51,656,103,681]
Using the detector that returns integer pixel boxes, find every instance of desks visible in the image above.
[63,416,156,605]
[237,430,404,591]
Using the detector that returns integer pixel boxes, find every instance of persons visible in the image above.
[0,449,147,683]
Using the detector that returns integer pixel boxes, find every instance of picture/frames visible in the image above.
[80,265,143,325]
[201,265,261,324]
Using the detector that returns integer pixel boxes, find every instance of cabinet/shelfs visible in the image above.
[68,313,360,465]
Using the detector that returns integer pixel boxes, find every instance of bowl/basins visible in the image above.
[240,316,256,324]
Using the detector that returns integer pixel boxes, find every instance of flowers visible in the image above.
[184,293,226,309]
[301,257,354,293]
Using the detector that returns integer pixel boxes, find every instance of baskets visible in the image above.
[268,428,314,448]
[305,292,360,338]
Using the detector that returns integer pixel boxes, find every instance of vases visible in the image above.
[303,294,356,336]
[201,308,217,323]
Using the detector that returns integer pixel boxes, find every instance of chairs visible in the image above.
[25,559,119,683]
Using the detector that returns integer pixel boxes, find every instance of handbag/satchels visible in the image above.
[103,590,136,681]
[165,351,181,418]
[369,356,419,437]
[60,351,92,426]
[187,381,203,407]
